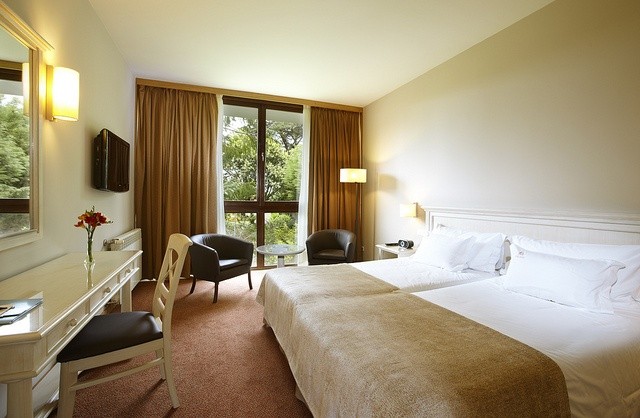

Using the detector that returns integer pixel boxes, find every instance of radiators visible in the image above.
[104,227,143,307]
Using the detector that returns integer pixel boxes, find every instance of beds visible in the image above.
[263,206,640,418]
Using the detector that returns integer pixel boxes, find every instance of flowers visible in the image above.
[75,205,114,262]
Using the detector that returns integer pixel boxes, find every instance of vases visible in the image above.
[83,238,95,264]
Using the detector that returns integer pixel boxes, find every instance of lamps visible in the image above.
[46,64,79,122]
[340,167,367,262]
[22,62,30,118]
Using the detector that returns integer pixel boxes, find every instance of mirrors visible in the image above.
[0,1,57,251]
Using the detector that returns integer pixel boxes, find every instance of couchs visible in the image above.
[305,229,356,266]
[188,233,254,304]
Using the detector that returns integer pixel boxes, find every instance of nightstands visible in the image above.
[376,243,417,259]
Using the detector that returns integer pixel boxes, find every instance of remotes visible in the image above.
[385,242,398,245]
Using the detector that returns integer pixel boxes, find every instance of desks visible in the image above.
[255,243,306,268]
[1,250,143,418]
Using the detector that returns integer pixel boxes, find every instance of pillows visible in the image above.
[432,223,507,274]
[503,243,626,315]
[412,228,481,273]
[501,235,640,313]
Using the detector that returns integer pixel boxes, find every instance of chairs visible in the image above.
[56,232,193,418]
